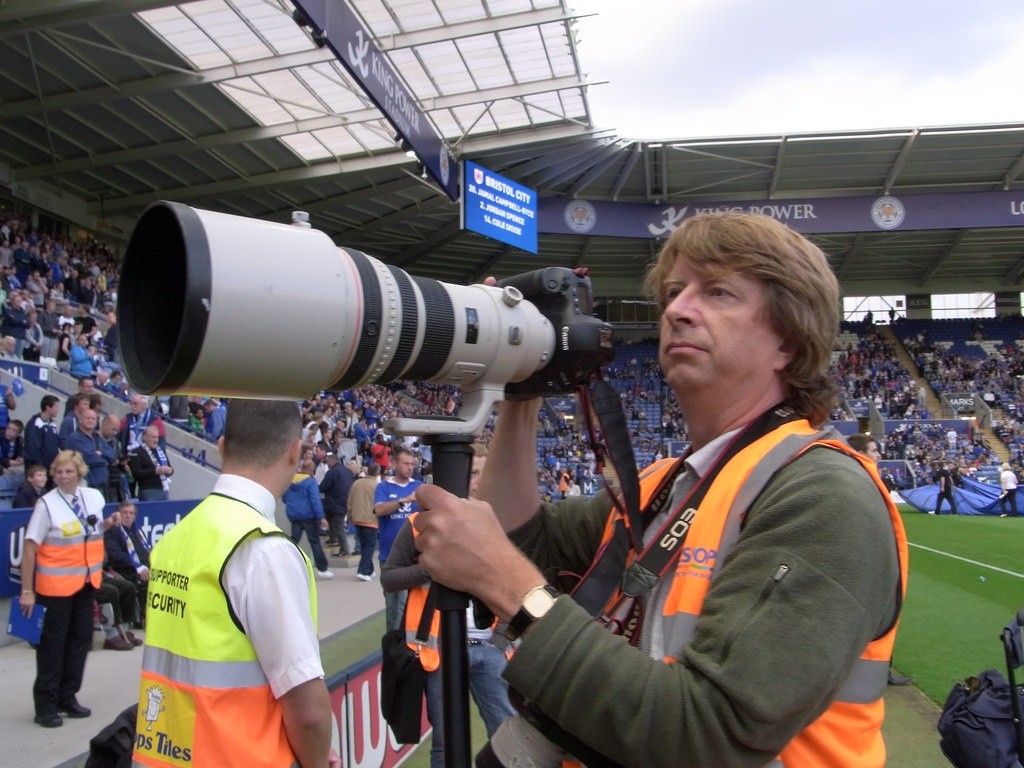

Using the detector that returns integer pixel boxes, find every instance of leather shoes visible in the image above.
[59,697,91,717]
[103,634,133,649]
[33,712,62,728]
[122,632,143,647]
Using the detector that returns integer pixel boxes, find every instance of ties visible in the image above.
[73,499,87,533]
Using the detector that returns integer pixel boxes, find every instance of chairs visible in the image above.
[2,218,1024,492]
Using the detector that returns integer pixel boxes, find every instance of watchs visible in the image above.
[505,585,562,641]
[397,498,406,510]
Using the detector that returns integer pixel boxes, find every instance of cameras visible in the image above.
[116,199,612,401]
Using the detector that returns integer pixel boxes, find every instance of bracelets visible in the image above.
[21,590,34,594]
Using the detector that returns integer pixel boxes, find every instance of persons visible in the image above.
[846,433,911,687]
[533,334,692,503]
[351,535,362,555]
[478,405,500,449]
[282,460,334,578]
[371,446,425,631]
[1,201,232,509]
[928,462,958,516]
[130,398,344,768]
[94,574,143,651]
[18,450,106,728]
[318,454,354,558]
[346,462,381,581]
[297,381,463,536]
[414,209,911,768]
[105,502,150,629]
[999,462,1018,517]
[827,307,1024,493]
[381,441,520,768]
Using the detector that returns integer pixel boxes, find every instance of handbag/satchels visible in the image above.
[374,627,426,745]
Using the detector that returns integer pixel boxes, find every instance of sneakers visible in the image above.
[318,569,334,579]
[356,573,371,581]
